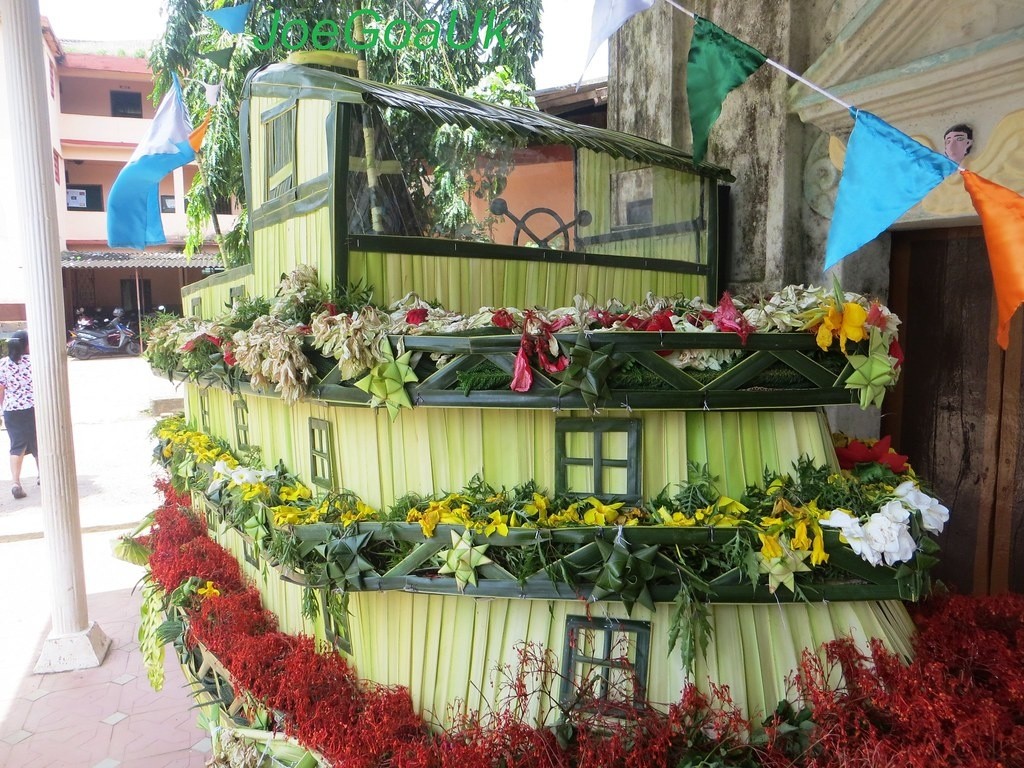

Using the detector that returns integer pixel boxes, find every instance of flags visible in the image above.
[107,72,199,251]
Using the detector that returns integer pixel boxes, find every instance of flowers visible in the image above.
[122,273,1024,768]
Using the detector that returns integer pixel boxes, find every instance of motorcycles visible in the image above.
[66,305,168,360]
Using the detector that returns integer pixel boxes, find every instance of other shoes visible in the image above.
[12,481,26,498]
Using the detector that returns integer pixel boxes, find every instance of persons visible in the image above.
[0,331,40,498]
